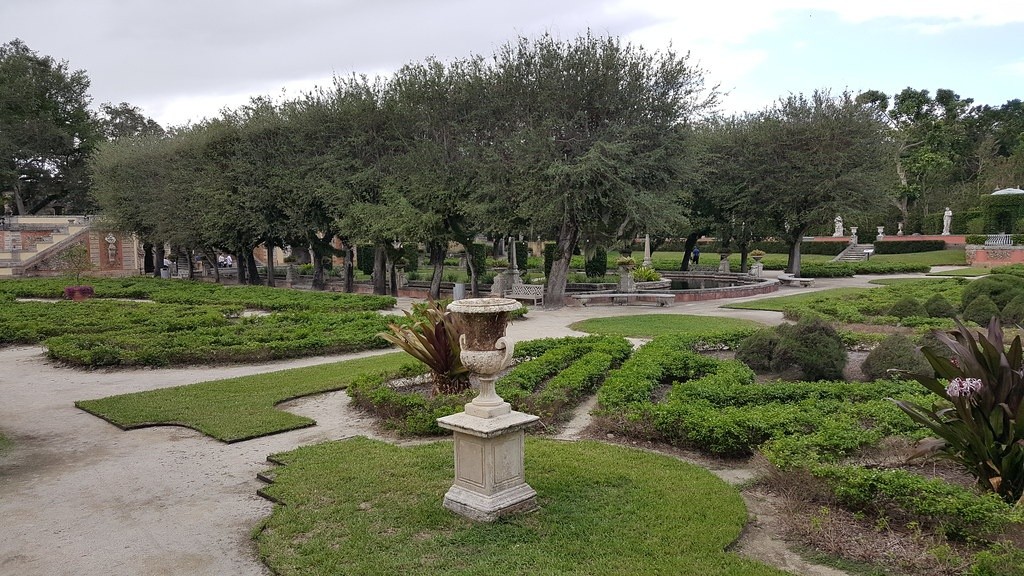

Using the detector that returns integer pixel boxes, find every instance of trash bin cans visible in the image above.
[452,282,465,301]
[160,267,171,278]
[202,263,211,275]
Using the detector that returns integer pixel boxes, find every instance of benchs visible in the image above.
[503,283,544,306]
[778,273,814,288]
[803,237,815,241]
[571,293,675,307]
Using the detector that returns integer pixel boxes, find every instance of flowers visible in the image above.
[64,285,94,296]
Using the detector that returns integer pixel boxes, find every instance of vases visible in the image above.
[72,291,87,301]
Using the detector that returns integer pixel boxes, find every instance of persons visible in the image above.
[164,257,171,270]
[834,215,843,234]
[218,252,234,268]
[195,254,200,261]
[943,207,952,234]
[692,246,699,263]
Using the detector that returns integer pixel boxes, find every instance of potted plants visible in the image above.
[619,247,632,257]
[395,257,410,272]
[875,222,885,237]
[850,222,858,237]
[614,256,637,271]
[716,248,732,258]
[749,249,767,261]
